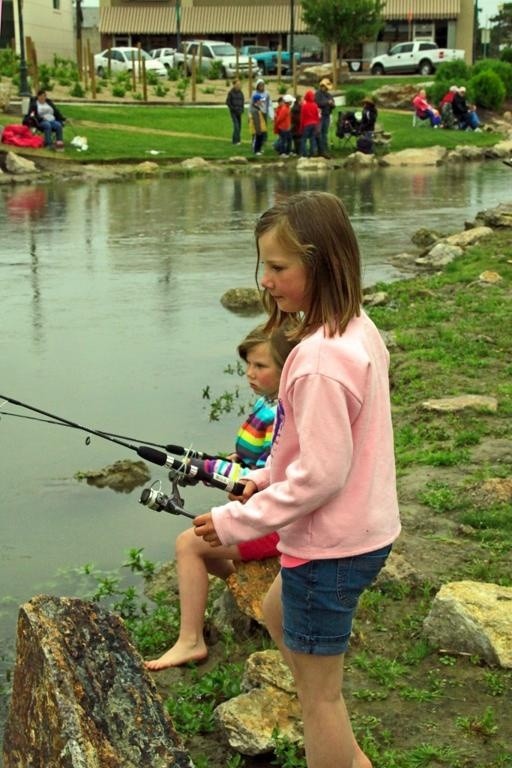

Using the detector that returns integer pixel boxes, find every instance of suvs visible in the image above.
[174,38,259,79]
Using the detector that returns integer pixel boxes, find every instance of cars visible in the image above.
[94,47,167,80]
[150,48,177,70]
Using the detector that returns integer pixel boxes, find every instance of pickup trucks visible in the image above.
[370,40,466,78]
[240,46,302,73]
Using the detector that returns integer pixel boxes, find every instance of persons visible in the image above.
[143,314,304,671]
[226,79,243,146]
[29,90,71,151]
[412,84,483,133]
[245,78,336,158]
[192,190,401,768]
[359,96,377,140]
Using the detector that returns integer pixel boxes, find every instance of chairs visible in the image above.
[339,111,392,155]
[443,103,462,129]
[411,106,438,127]
[21,97,65,144]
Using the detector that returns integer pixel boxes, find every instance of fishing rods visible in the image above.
[1,408,235,462]
[1,394,251,520]
